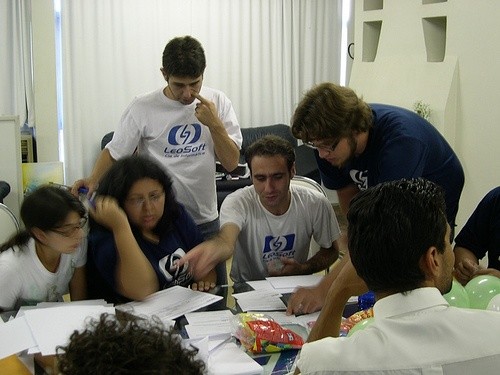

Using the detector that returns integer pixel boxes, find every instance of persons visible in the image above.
[284,82,465,317]
[0,181,88,313]
[84,154,218,304]
[283,176,500,375]
[452,186,500,285]
[175,134,342,283]
[67,35,244,310]
[56,312,206,374]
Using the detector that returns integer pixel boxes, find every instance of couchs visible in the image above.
[101,124,322,213]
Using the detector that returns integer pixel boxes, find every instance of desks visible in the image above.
[0,284,360,375]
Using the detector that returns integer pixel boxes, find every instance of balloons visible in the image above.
[442,275,500,313]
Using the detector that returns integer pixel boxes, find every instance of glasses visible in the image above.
[47,217,88,237]
[304,137,342,152]
